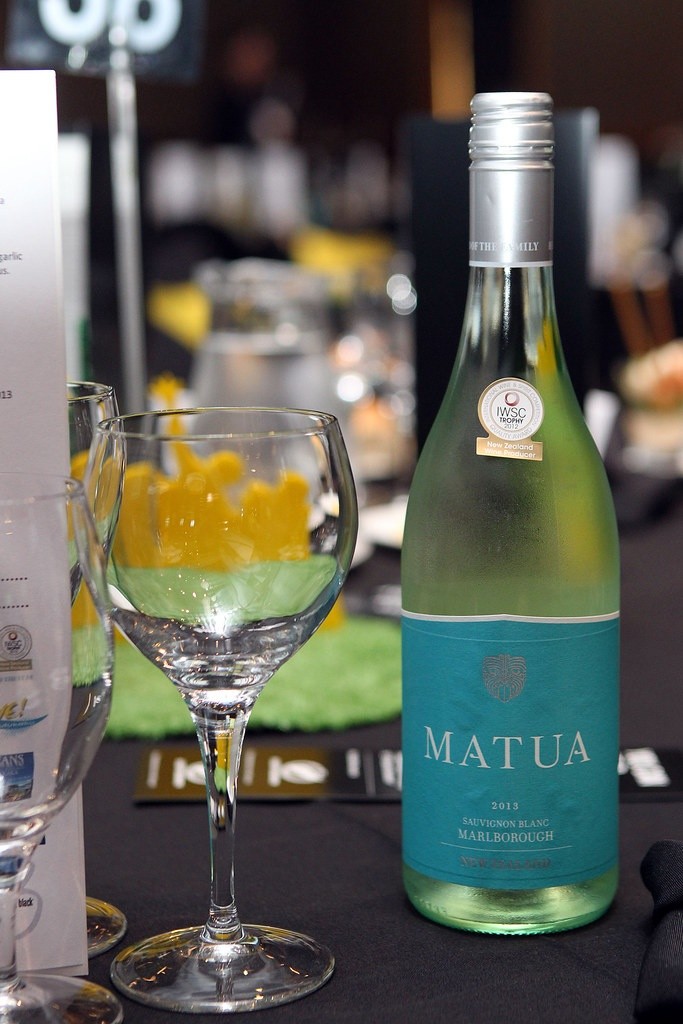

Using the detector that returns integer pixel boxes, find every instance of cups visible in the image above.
[173,256,348,526]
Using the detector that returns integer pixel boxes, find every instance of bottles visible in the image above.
[396,89,621,939]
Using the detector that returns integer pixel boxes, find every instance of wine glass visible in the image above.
[82,405,360,1013]
[1,472,124,1023]
[67,382,132,953]
